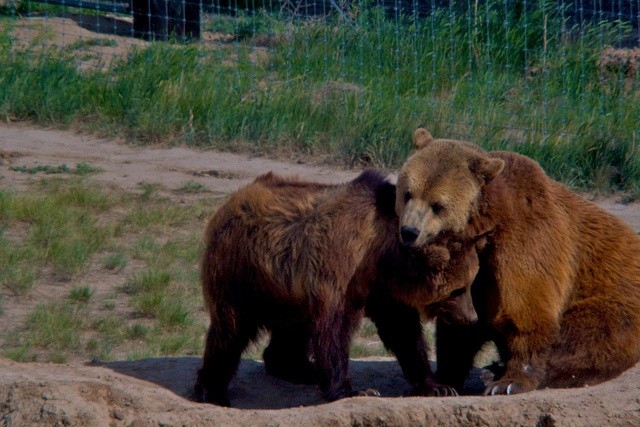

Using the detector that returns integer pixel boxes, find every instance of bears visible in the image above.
[187,171,480,408]
[394,127,640,398]
